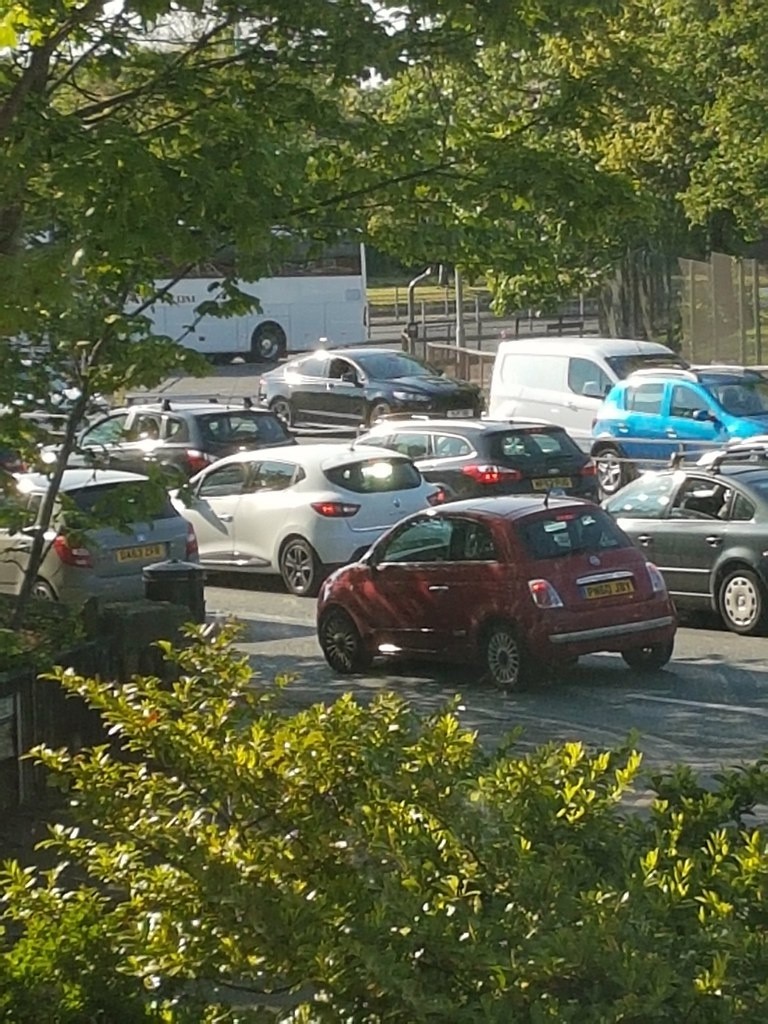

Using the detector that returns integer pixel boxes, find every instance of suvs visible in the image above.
[41,395,298,478]
[347,414,600,507]
[590,365,768,495]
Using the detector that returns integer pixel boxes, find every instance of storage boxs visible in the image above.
[142,556,208,628]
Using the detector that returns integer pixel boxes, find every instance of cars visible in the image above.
[0,386,109,438]
[599,446,768,635]
[168,443,446,599]
[258,346,484,430]
[316,491,681,691]
[0,469,199,613]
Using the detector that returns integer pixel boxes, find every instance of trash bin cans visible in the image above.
[140,558,207,624]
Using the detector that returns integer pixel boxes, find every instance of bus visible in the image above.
[7,221,371,365]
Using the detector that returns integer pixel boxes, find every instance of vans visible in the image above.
[487,334,688,456]
